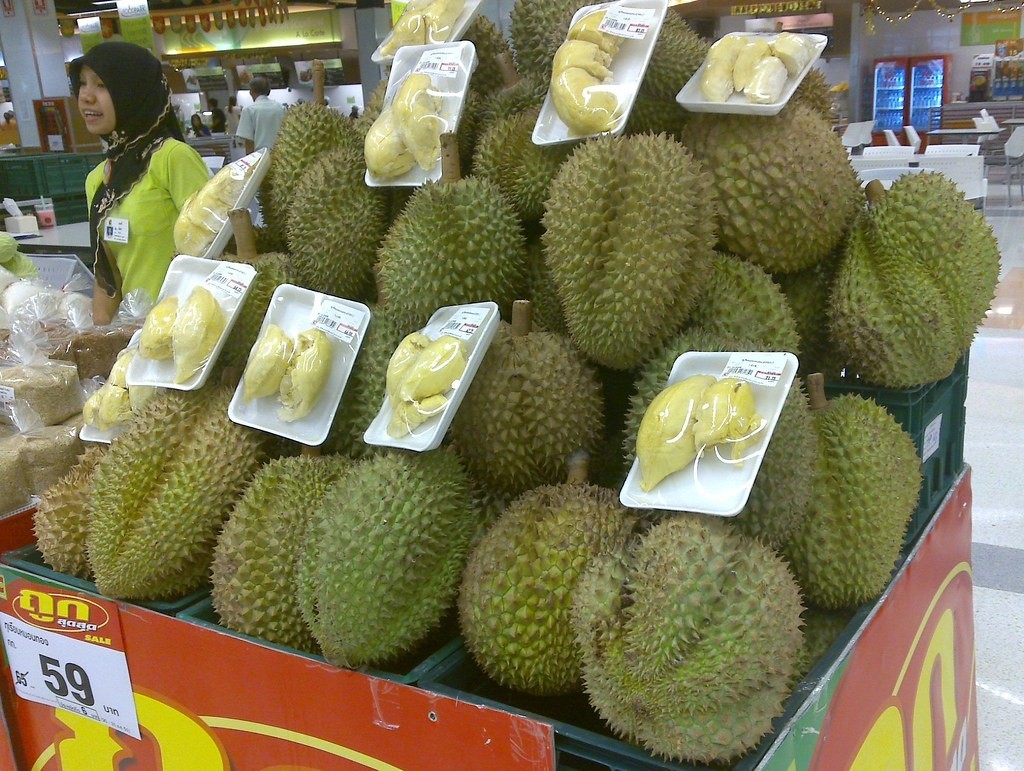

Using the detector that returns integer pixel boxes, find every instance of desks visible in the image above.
[16,221,91,254]
[925,128,1007,144]
[1001,118,1024,185]
[847,153,972,168]
[861,179,987,216]
[830,123,848,132]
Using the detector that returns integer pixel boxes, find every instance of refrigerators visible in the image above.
[873,54,952,147]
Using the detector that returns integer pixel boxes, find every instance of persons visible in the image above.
[349,105,359,119]
[208,97,227,133]
[190,114,211,137]
[0,110,21,147]
[234,77,287,155]
[224,96,243,134]
[68,41,215,324]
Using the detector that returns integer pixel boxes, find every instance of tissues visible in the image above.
[3,198,39,238]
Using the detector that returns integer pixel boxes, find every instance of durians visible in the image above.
[29,0,1004,768]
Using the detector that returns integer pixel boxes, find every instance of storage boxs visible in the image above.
[0,151,109,231]
[0,343,972,771]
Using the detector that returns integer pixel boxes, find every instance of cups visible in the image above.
[34,204,54,227]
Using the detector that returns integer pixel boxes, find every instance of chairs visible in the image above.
[842,120,1024,207]
